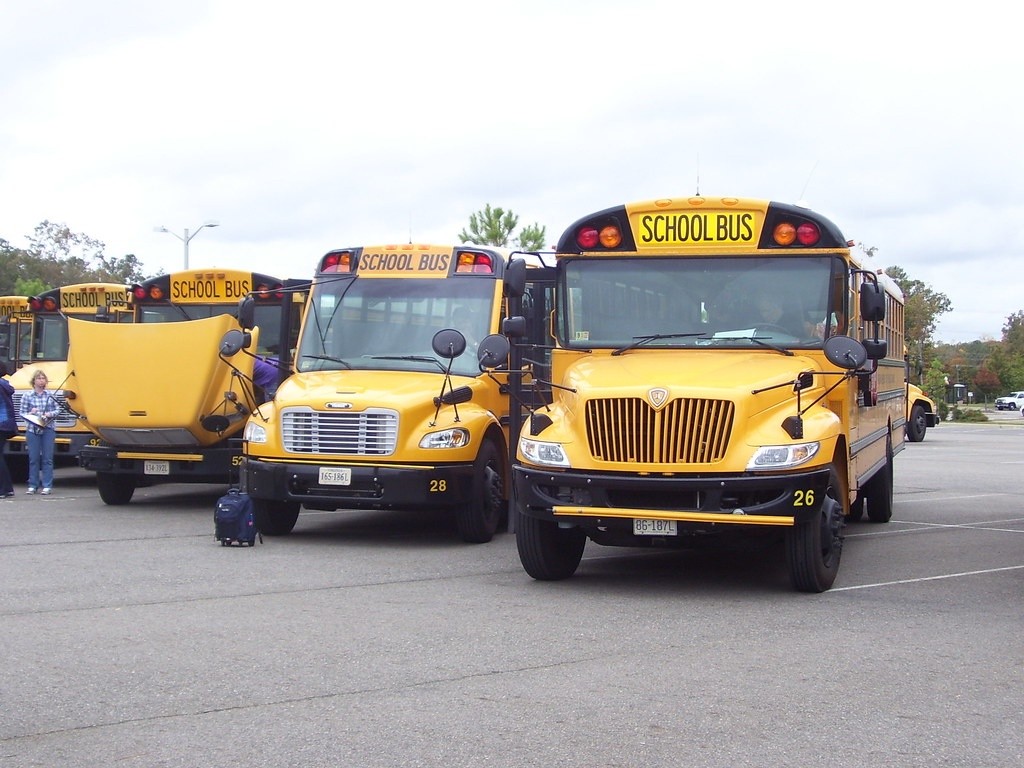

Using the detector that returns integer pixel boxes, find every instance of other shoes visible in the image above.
[7,492,15,497]
[0,495,6,499]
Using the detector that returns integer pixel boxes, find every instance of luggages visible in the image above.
[213,437,263,547]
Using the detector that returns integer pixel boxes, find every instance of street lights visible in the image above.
[154,224,218,272]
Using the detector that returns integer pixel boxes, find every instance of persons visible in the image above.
[452,306,479,357]
[0,361,17,499]
[19,371,60,496]
[743,283,804,340]
[253,356,278,404]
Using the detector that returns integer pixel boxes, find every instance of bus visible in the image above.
[219,246,555,543]
[0,282,147,482]
[65,270,312,505]
[477,197,909,593]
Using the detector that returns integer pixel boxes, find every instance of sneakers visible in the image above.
[41,488,51,495]
[26,487,37,494]
